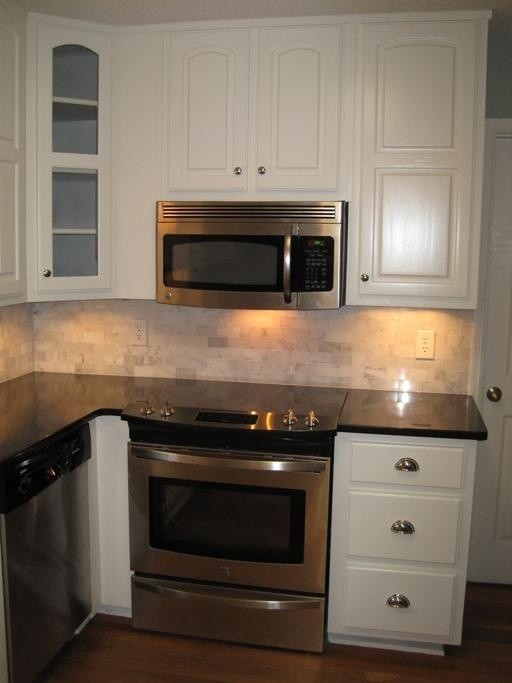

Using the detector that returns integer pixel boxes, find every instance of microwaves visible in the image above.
[156,200,347,311]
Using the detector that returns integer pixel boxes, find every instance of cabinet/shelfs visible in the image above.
[159,14,354,200]
[0,10,26,308]
[326,434,485,655]
[23,11,112,303]
[339,10,489,309]
[114,27,160,302]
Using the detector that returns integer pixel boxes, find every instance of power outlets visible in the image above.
[414,330,437,360]
[130,319,148,345]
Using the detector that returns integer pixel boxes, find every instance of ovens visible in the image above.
[127,438,332,652]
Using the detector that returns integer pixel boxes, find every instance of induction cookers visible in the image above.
[120,377,349,432]
